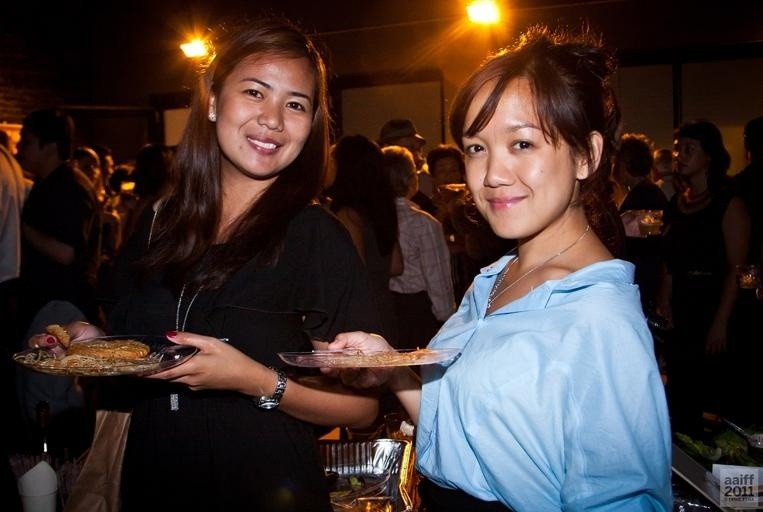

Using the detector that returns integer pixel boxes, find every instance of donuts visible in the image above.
[68,339,150,359]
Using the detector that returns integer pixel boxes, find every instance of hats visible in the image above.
[378,118,428,145]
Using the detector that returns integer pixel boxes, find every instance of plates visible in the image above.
[13,334,197,376]
[320,462,390,499]
[277,348,459,369]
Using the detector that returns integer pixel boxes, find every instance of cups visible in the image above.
[345,412,411,441]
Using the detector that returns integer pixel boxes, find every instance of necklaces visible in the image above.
[485,224,595,311]
[166,281,206,413]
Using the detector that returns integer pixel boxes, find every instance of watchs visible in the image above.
[257,366,287,412]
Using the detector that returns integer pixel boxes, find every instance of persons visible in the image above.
[25,16,390,510]
[307,18,676,512]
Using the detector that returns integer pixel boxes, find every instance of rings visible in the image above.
[31,334,40,350]
